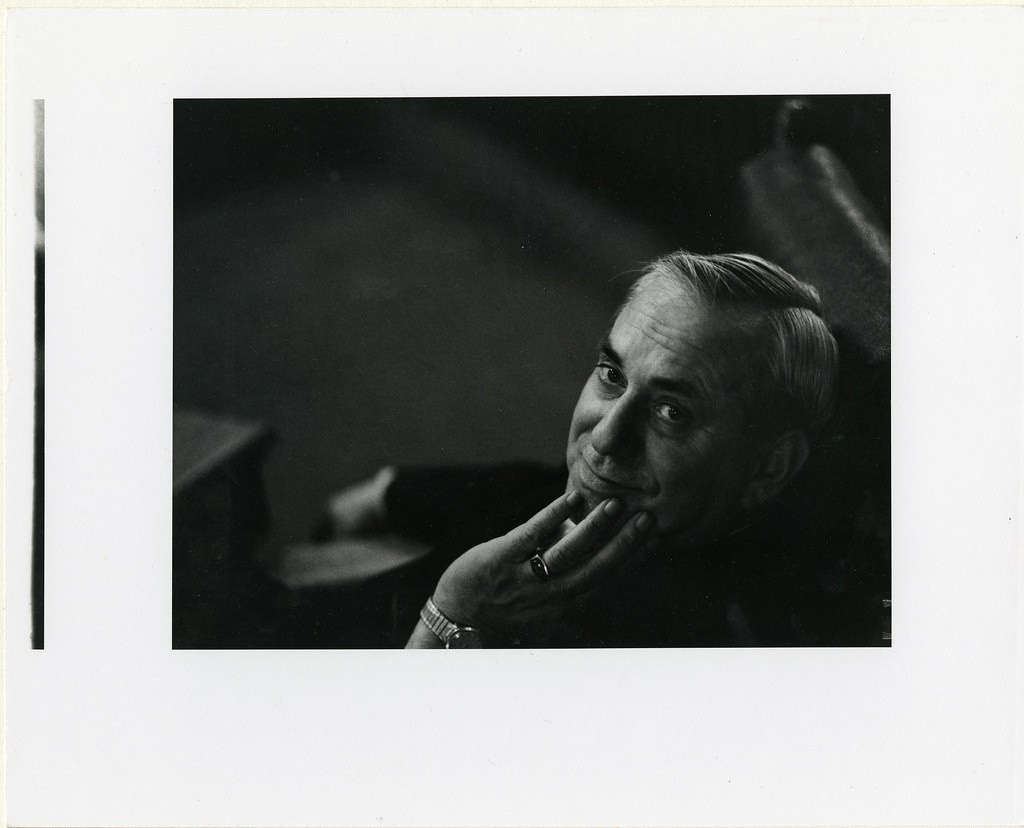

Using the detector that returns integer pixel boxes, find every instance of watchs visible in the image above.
[419,596,486,649]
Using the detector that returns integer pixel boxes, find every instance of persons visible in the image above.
[402,251,839,649]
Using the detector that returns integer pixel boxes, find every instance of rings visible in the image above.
[529,553,552,582]
